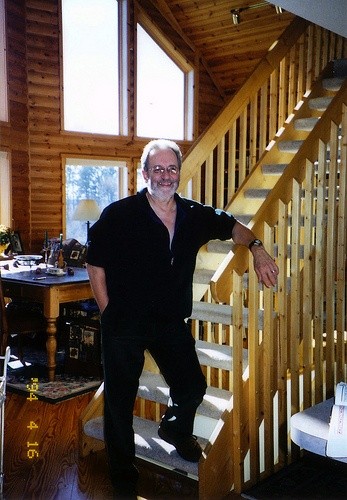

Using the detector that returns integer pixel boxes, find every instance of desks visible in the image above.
[2,268,100,383]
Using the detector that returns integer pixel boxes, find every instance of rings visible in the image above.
[272,270,275,273]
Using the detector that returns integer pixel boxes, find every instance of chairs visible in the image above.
[0,278,66,371]
[288,381,347,463]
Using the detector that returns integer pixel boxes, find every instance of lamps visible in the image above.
[72,198,101,243]
[231,13,242,25]
[275,6,283,14]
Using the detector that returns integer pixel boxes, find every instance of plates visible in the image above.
[45,272,67,276]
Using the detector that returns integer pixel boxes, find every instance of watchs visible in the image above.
[249,239,263,251]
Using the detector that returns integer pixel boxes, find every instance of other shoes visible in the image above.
[158,426,203,462]
[112,460,141,495]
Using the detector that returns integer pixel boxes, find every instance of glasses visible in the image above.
[147,165,179,174]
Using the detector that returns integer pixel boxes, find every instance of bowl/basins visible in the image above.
[47,268,63,273]
[14,255,43,265]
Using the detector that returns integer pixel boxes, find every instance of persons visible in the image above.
[87,139,280,500]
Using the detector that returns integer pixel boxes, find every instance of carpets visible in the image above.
[2,347,102,403]
